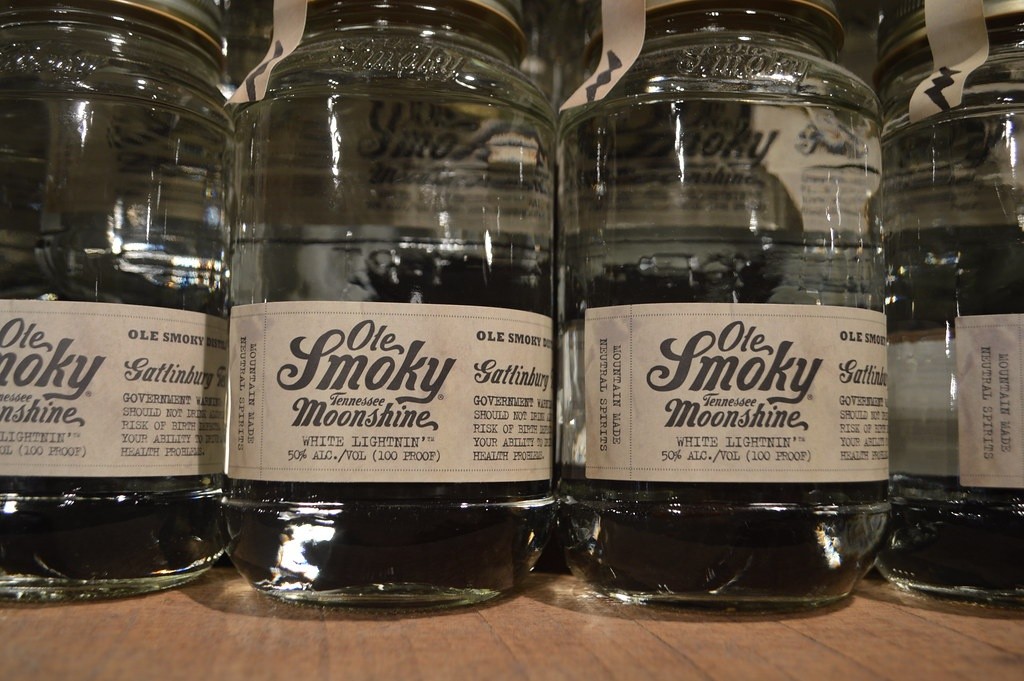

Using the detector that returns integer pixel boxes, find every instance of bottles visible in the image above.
[880,0,1024,600]
[557,0,881,610]
[0,0,233,602]
[226,0,555,603]
